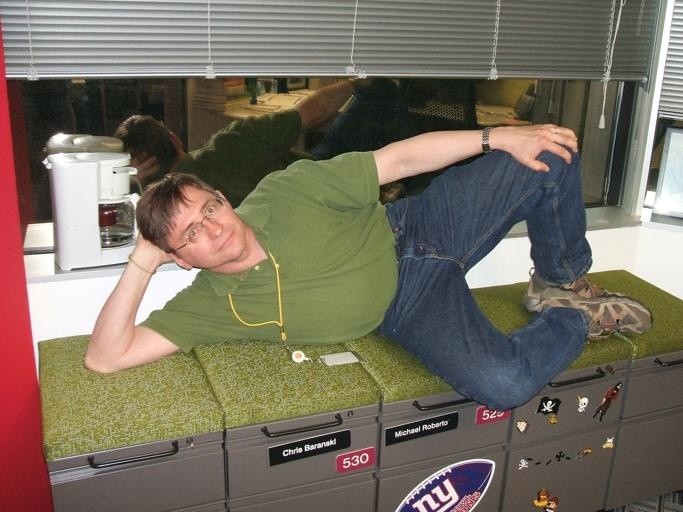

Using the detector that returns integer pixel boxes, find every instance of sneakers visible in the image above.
[522,268,624,314]
[538,294,652,342]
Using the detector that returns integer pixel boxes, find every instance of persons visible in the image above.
[593,382,622,421]
[82,122,653,411]
[113,77,429,209]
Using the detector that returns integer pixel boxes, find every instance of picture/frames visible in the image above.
[651,128,683,219]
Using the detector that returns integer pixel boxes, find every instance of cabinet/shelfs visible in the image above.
[45,351,683,512]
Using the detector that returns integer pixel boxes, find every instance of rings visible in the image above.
[554,126,558,134]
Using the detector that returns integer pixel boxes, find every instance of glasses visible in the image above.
[175,194,224,252]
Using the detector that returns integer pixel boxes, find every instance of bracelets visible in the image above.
[482,125,491,154]
[349,79,363,94]
[127,253,156,275]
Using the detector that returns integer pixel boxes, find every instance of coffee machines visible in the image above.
[43,132,124,152]
[41,152,143,274]
